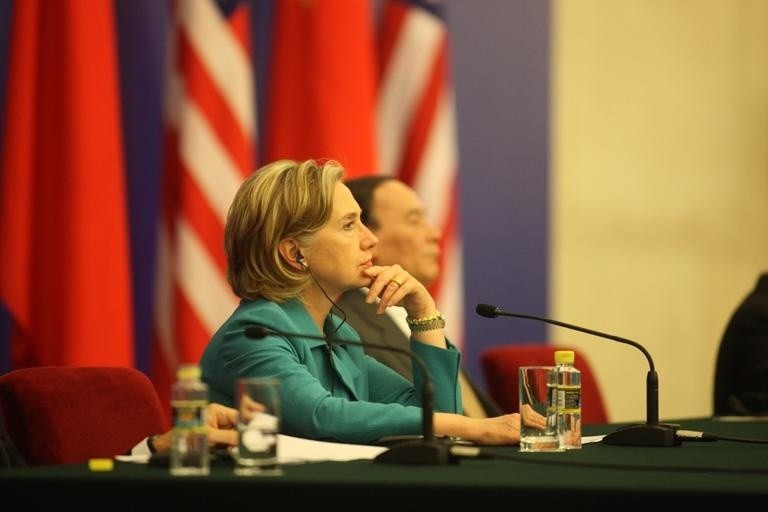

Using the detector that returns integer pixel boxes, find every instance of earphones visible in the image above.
[294,253,347,396]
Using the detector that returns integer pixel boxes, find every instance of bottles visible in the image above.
[546,352,582,449]
[170,368,209,475]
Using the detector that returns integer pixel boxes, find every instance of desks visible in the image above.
[0,418,768,512]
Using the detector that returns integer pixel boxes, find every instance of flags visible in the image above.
[153,1,259,376]
[379,4,467,357]
[0,0,133,369]
[266,1,379,179]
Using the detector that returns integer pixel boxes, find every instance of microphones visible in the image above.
[241,325,453,466]
[475,303,680,448]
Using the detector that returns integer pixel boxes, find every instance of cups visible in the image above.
[517,366,566,456]
[234,379,286,481]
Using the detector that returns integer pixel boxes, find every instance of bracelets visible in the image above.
[405,311,447,332]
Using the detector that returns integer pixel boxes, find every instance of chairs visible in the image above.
[480,346,608,425]
[0,368,169,463]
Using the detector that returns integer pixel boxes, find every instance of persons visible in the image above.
[199,159,521,447]
[331,176,507,420]
[713,274,767,416]
[0,301,241,465]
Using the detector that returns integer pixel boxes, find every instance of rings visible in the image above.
[391,277,404,285]
